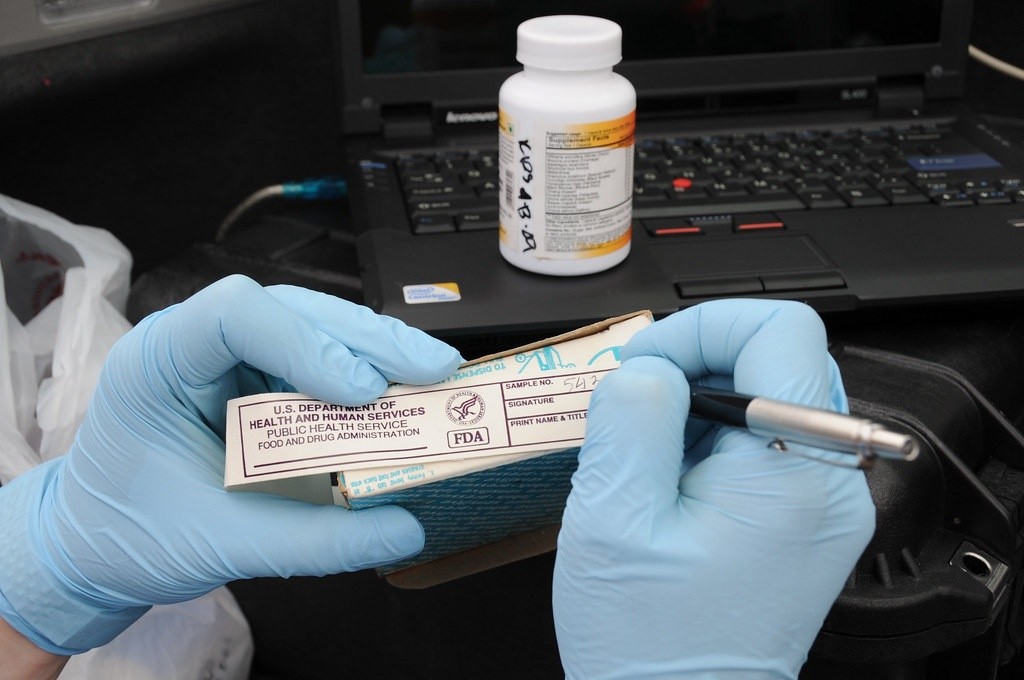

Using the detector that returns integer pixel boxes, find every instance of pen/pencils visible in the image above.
[689,384,919,461]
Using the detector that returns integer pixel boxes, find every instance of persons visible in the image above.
[0,274,877,680]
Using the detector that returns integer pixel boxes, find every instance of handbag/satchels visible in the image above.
[0,189,255,679]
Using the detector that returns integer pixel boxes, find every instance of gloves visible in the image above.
[553,297,876,680]
[0,273,464,657]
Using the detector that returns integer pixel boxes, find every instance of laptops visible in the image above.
[332,0,1024,337]
